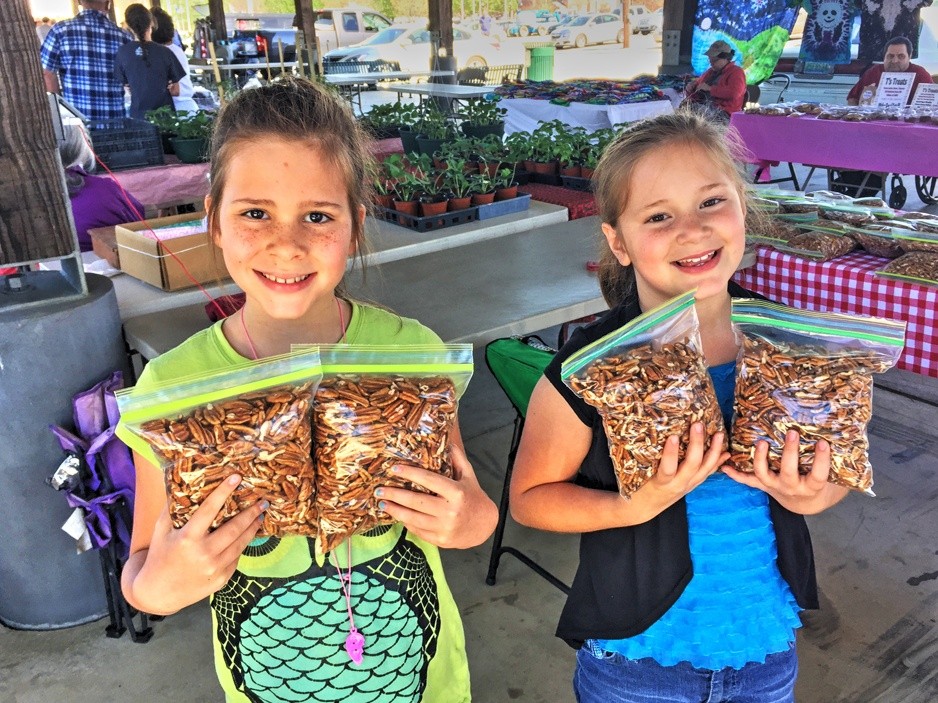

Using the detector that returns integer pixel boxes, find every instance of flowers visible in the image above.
[144,107,212,142]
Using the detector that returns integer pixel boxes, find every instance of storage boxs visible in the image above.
[518,170,560,186]
[562,175,595,192]
[372,205,479,234]
[479,191,531,220]
[114,214,228,292]
[87,117,166,176]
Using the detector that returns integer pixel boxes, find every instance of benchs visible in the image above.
[460,63,524,91]
[323,61,413,100]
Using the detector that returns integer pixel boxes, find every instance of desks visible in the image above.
[103,141,402,214]
[189,64,677,147]
[129,215,756,358]
[730,245,938,378]
[39,199,568,322]
[724,112,938,192]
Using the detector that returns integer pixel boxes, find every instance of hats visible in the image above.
[704,40,731,57]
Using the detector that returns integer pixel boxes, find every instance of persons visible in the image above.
[29,0,199,129]
[509,114,852,703]
[678,41,747,126]
[120,79,500,702]
[832,36,935,199]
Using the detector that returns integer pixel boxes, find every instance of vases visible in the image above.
[168,135,205,163]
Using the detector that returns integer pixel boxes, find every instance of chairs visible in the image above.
[483,337,572,594]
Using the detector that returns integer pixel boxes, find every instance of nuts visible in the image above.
[305,375,456,553]
[727,333,888,494]
[569,337,729,496]
[142,381,318,540]
[743,100,883,121]
[742,192,937,283]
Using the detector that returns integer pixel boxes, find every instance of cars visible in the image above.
[549,13,634,49]
[190,0,663,88]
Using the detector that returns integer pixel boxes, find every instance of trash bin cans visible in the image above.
[0,270,137,632]
[522,40,557,82]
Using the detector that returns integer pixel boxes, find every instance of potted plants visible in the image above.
[354,101,601,225]
